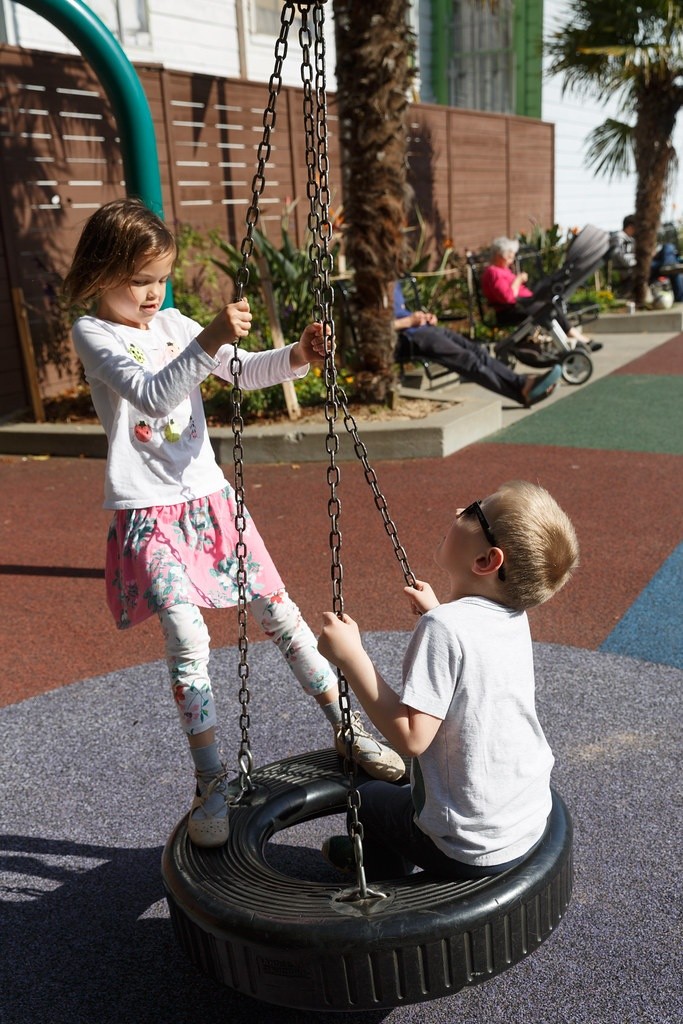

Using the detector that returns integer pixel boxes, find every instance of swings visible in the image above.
[150,1,575,1012]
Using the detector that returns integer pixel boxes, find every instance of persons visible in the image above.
[61,200,406,845]
[313,481,578,877]
[387,214,683,408]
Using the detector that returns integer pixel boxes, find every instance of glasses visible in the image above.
[461,498,507,582]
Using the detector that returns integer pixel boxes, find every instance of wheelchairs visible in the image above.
[461,224,622,385]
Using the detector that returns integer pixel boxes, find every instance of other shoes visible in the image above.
[188,766,231,845]
[335,712,407,782]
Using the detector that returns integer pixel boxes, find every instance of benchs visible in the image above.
[333,274,453,380]
[606,223,683,302]
[466,244,599,343]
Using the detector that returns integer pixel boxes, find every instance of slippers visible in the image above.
[524,383,556,408]
[529,365,562,397]
